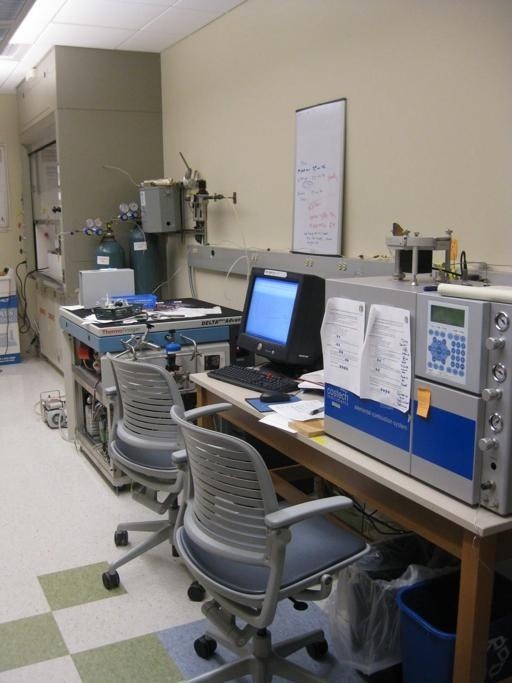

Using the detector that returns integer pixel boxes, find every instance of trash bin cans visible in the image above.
[340,533,511,682]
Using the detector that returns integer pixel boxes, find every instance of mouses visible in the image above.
[259,391,292,402]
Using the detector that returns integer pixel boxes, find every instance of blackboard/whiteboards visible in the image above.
[291,98,346,257]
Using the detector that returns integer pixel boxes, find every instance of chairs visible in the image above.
[100,348,236,606]
[165,396,370,683]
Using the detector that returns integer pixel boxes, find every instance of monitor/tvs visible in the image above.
[237,267,325,377]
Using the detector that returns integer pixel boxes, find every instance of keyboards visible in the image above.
[207,365,297,392]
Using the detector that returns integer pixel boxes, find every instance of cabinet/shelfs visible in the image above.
[29,277,79,377]
[58,293,257,498]
[16,43,163,142]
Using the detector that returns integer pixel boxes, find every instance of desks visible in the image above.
[184,360,512,681]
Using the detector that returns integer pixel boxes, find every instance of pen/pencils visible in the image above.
[311,407,323,415]
[162,315,186,317]
